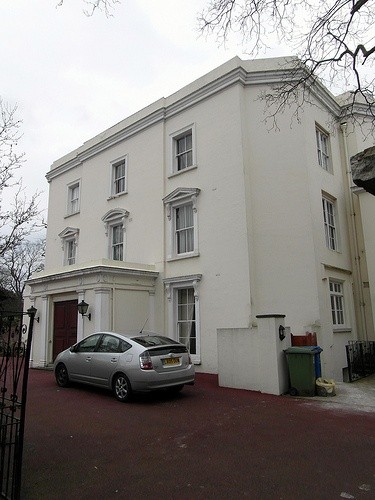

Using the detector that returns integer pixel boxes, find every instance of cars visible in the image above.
[52,330,196,400]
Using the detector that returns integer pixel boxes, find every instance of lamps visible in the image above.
[27,306,39,323]
[77,300,91,321]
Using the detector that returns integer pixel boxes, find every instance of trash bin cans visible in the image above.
[304,345,324,380]
[283,347,316,396]
